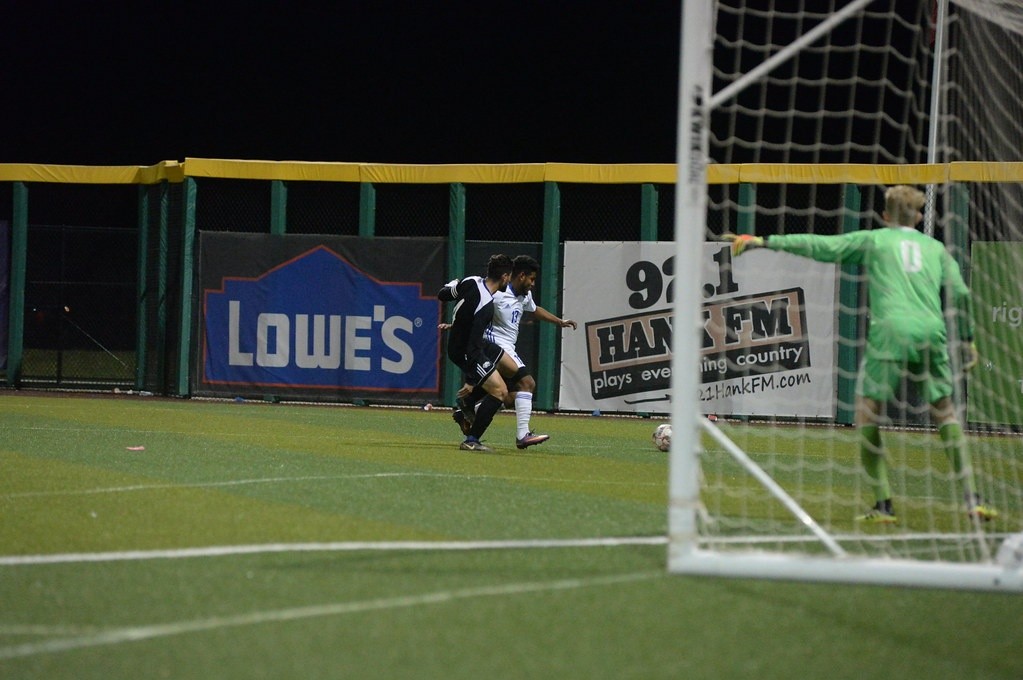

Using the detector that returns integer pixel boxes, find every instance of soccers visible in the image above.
[652,423,674,452]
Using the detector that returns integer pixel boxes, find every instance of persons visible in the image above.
[437,255,518,453]
[720,185,999,525]
[437,256,577,450]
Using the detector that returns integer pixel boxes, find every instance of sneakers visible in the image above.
[453,411,471,436]
[854,508,898,524]
[453,397,476,424]
[459,440,492,451]
[965,492,998,522]
[516,428,550,449]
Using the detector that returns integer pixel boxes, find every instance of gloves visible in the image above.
[961,341,978,372]
[719,233,764,257]
